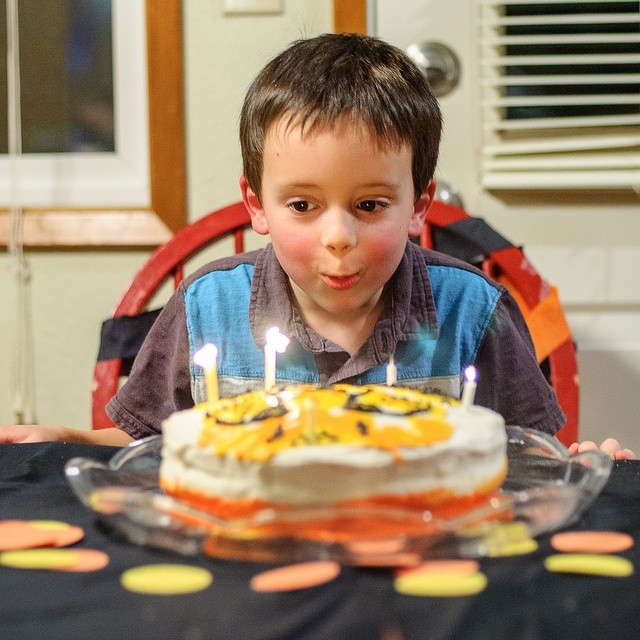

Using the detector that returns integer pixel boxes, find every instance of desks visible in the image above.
[0,443,638,640]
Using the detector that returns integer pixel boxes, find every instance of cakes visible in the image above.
[157,343,510,538]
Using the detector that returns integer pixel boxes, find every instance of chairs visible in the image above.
[91,195,582,452]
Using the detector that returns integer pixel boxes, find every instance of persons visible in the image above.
[0,32,638,469]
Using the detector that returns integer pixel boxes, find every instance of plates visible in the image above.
[61,426,614,570]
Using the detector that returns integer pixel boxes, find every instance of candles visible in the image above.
[262,319,289,392]
[386,350,403,387]
[460,363,480,406]
[194,342,220,399]
